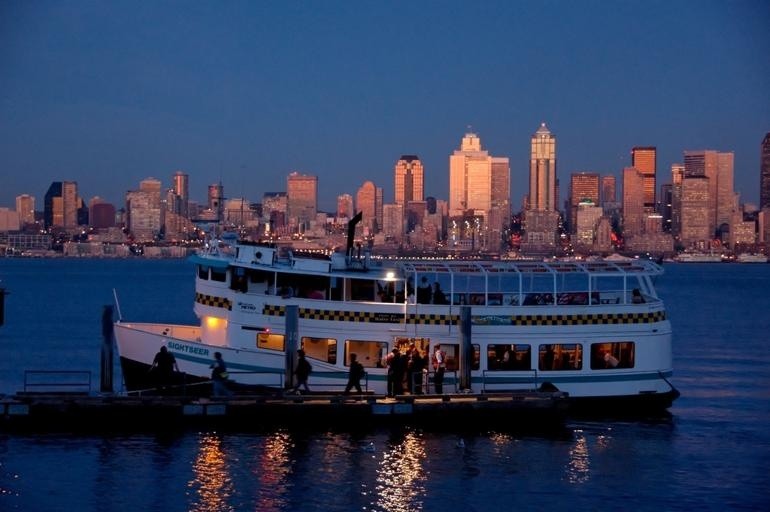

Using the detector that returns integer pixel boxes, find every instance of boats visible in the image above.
[113,211,681,412]
[674,251,767,264]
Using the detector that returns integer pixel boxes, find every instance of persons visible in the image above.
[542,344,555,370]
[275,280,294,298]
[417,276,433,303]
[343,354,363,396]
[385,344,446,398]
[433,281,449,304]
[631,289,645,304]
[501,345,516,370]
[210,352,236,397]
[232,277,247,293]
[148,346,181,396]
[292,350,311,394]
[555,352,574,369]
